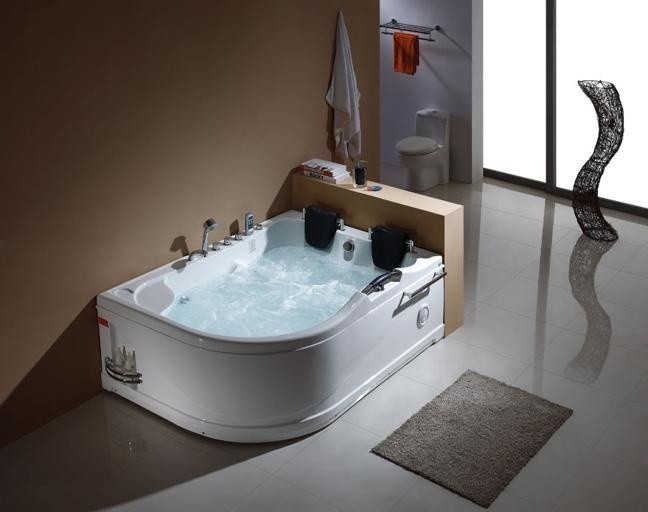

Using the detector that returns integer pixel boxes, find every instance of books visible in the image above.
[302,171,352,184]
[300,158,347,177]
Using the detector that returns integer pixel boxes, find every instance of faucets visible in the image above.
[189,249,208,261]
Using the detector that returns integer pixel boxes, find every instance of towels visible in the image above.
[393,31,419,75]
[325,12,362,175]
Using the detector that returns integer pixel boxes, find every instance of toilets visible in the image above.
[397,108,451,193]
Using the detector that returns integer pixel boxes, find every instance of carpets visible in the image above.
[371,367,575,509]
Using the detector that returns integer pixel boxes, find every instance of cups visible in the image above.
[352,165,368,188]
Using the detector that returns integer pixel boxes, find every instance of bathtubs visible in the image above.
[94,208,445,444]
